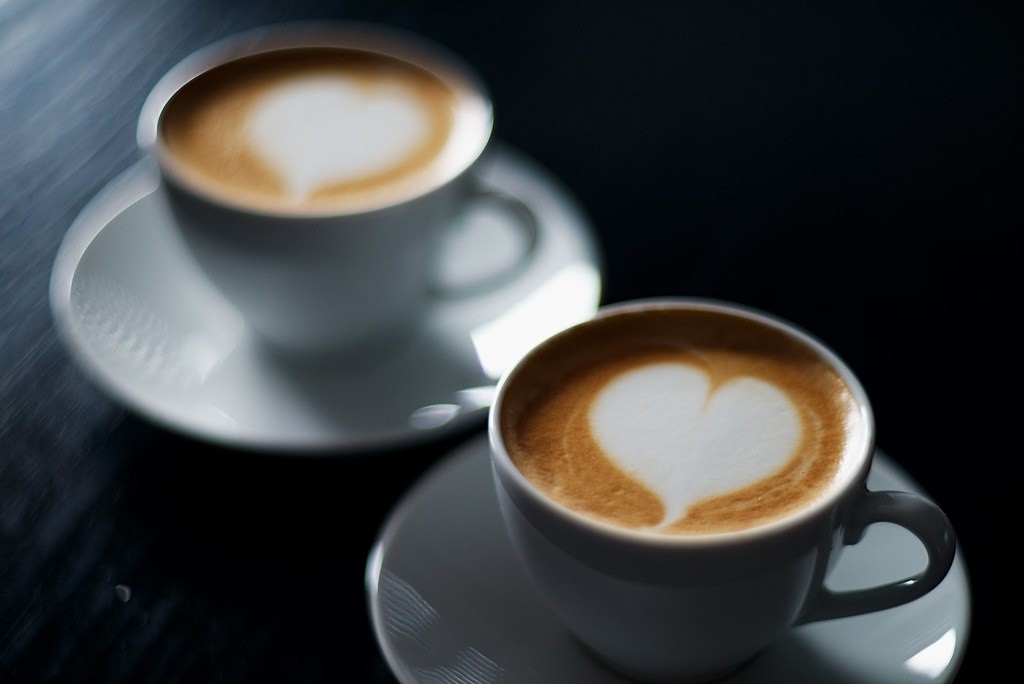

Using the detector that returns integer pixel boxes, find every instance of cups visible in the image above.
[490,301,958,679]
[137,28,542,363]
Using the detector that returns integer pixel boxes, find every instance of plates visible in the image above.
[47,155,604,448]
[365,439,970,684]
